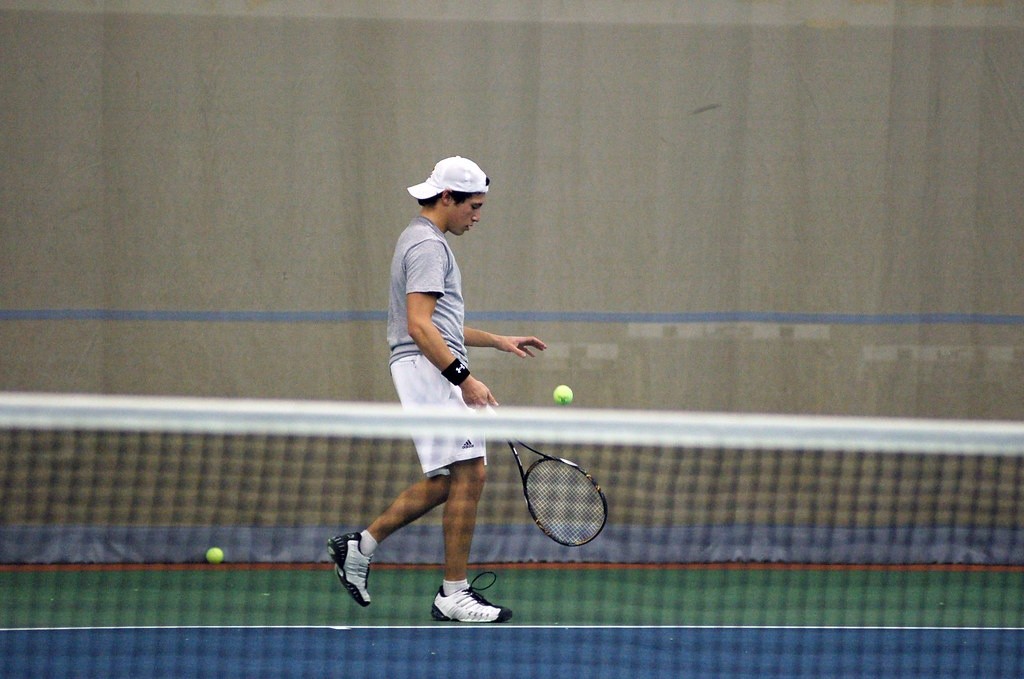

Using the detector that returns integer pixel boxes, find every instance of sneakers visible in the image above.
[431,573,513,623]
[328,533,373,607]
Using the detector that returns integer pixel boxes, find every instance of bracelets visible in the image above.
[442,359,470,386]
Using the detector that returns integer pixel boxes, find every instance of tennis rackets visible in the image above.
[486,405,608,548]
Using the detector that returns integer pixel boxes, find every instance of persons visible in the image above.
[329,157,546,623]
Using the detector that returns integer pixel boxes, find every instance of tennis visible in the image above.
[206,547,224,564]
[553,385,573,405]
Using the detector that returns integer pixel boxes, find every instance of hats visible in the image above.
[407,156,490,199]
